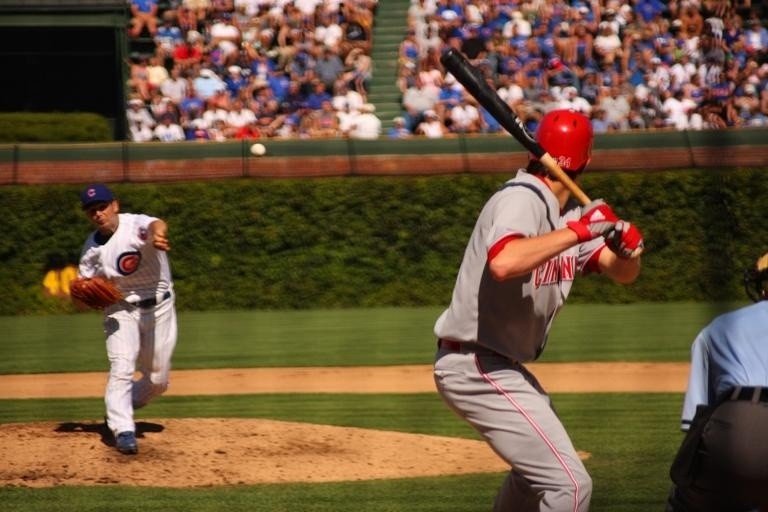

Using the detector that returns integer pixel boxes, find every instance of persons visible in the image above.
[125,0,382,142]
[69,182,179,456]
[428,106,647,511]
[391,2,768,138]
[661,242,767,512]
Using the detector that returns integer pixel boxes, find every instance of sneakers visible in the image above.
[116,429,137,454]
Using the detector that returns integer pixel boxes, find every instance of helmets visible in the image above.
[744,252,768,303]
[527,109,594,172]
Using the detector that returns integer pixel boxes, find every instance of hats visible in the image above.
[81,183,113,211]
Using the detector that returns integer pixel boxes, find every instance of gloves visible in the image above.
[603,218,645,260]
[566,198,618,245]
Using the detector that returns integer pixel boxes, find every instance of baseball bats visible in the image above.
[441,48,642,258]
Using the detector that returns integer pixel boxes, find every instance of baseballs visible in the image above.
[251,144,266,157]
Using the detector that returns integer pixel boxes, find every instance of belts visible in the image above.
[132,291,171,309]
[436,337,479,351]
[715,385,768,405]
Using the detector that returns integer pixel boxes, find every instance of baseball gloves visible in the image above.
[71,278,121,309]
